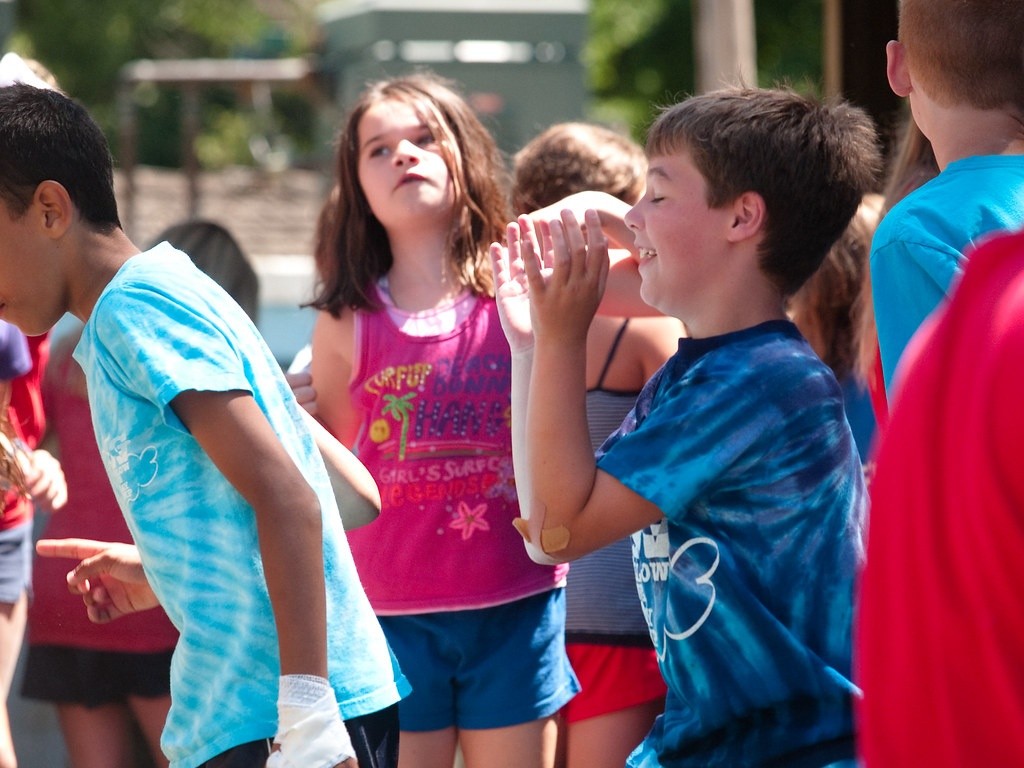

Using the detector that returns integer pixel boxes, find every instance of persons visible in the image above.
[1,0,1024,768]
